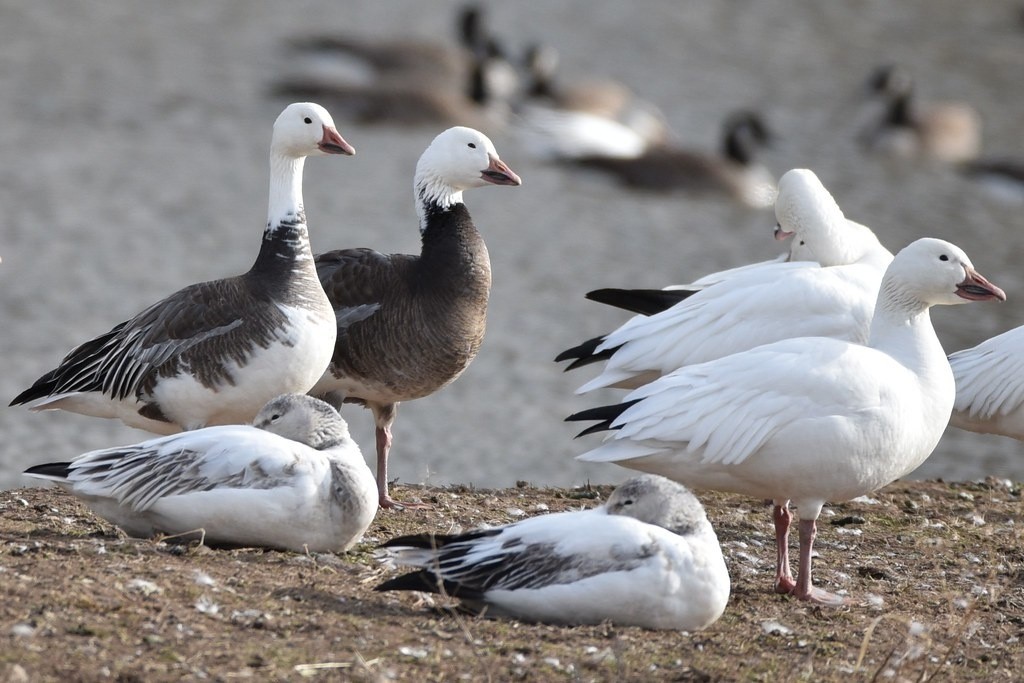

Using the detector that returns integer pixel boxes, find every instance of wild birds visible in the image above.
[553,169,896,398]
[22,393,381,555]
[316,127,523,509]
[7,3,1023,210]
[562,237,1007,611]
[369,475,731,632]
[947,323,1024,442]
[6,100,358,435]
